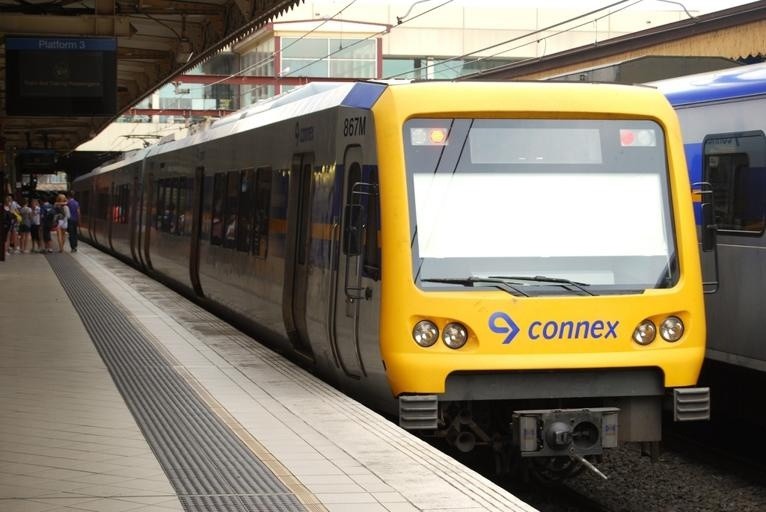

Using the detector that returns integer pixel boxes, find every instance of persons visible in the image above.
[156,202,245,248]
[0,190,81,262]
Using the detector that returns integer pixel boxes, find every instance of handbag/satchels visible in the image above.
[56,208,64,220]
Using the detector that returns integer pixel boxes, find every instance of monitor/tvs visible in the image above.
[16,148,57,174]
[4,34,118,116]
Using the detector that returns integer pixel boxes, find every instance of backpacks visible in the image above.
[43,207,58,228]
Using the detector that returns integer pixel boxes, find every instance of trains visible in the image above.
[72,81,721,489]
[535,55,765,434]
